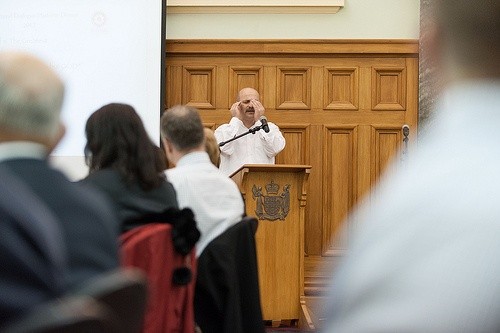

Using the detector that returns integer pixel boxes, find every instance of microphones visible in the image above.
[259,115,269,133]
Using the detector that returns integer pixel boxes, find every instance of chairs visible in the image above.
[8,216,258,333]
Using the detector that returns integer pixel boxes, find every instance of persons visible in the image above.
[203,127,220,167]
[160,104,245,259]
[75,103,178,237]
[214,88,286,178]
[0,48,121,333]
[318,0,500,333]
